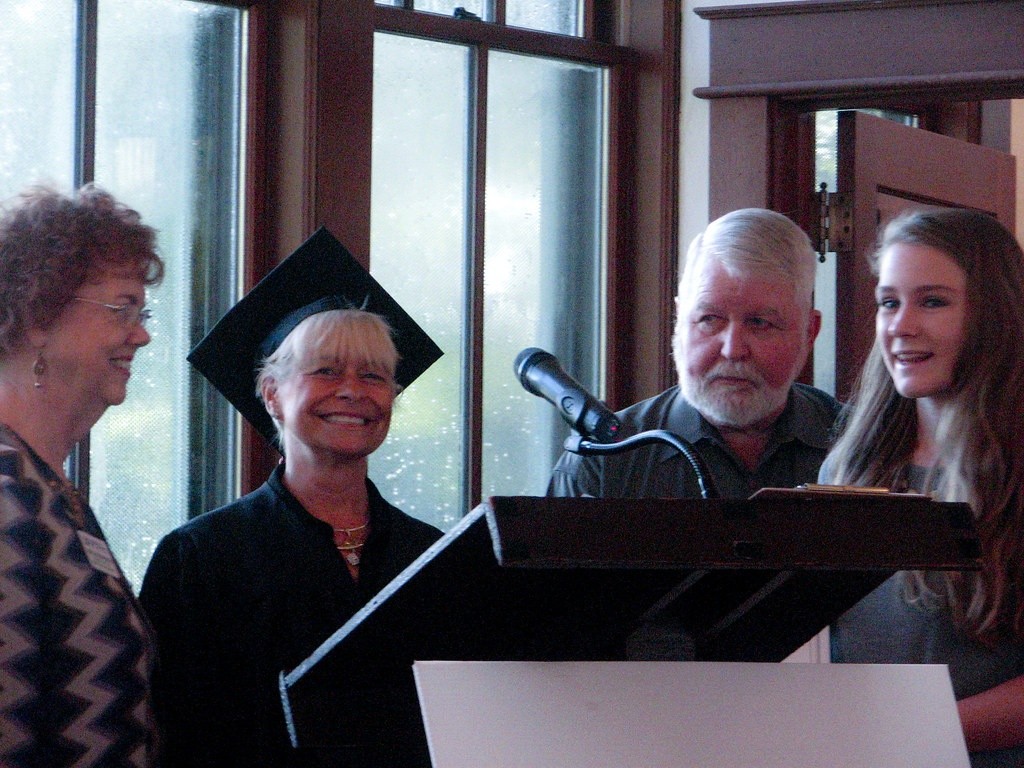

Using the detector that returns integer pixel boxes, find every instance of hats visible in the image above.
[185,224,445,461]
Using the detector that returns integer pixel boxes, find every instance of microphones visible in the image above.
[515,347,619,443]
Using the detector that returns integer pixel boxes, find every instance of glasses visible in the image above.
[70,296,155,330]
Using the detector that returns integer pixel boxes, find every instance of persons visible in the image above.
[819,208,1024,768]
[0,186,171,768]
[544,208,847,503]
[138,226,446,768]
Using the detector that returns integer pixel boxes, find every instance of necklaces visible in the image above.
[333,521,368,566]
